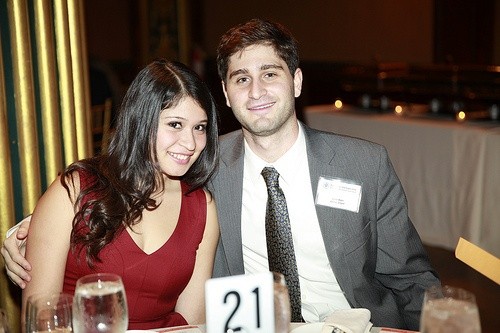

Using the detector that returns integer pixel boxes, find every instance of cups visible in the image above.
[26,290,72,333]
[273,271,291,333]
[73,272,128,333]
[419,286,482,333]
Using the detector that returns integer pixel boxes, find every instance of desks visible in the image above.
[153,322,420,333]
[303,102,500,257]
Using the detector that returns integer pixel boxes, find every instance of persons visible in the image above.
[0,17,442,331]
[20,57,220,333]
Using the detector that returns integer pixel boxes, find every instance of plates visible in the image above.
[291,321,324,333]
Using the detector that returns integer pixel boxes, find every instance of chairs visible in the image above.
[91,98,113,158]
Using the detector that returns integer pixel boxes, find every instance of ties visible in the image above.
[261,166,303,323]
